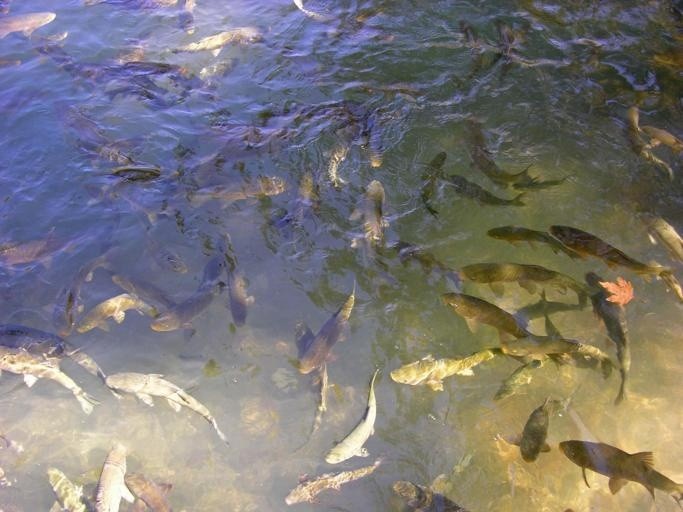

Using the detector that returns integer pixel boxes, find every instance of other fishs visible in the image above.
[0,1,682,508]
